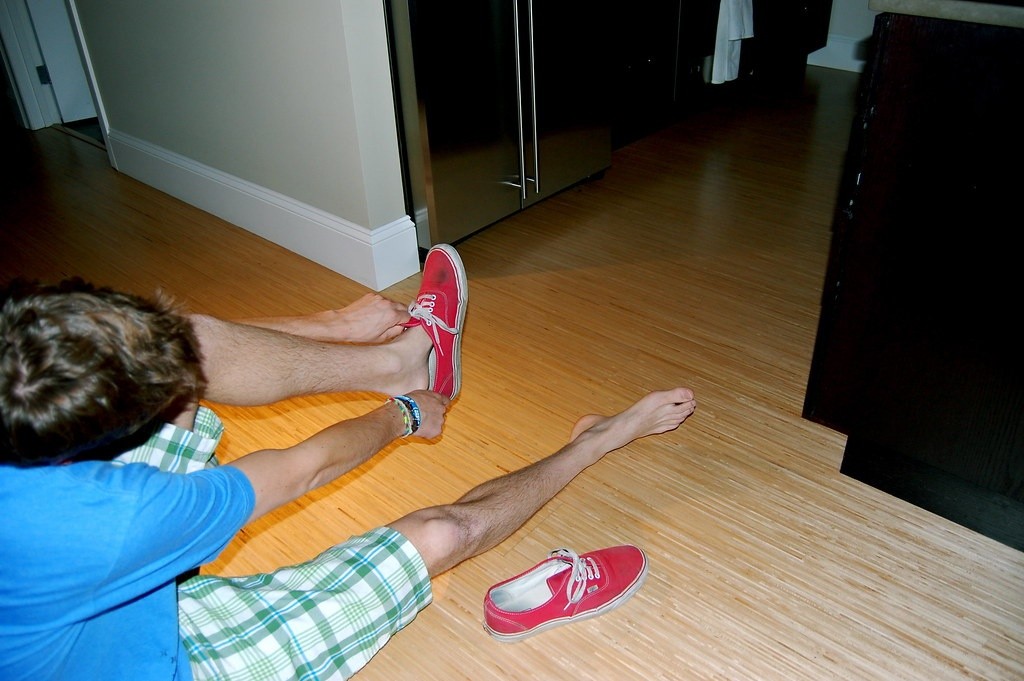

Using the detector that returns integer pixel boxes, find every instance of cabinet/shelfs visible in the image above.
[798,10,1024,558]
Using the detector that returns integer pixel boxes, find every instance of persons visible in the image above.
[0,239,697,680]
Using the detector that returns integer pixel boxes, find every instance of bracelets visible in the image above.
[383,392,422,438]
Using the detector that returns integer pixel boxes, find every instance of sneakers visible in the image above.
[400,243,469,404]
[484,545,647,640]
